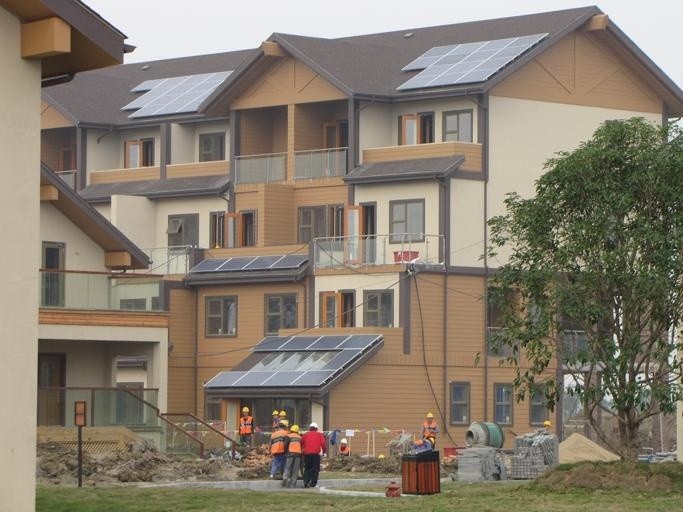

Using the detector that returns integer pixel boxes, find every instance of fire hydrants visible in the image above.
[385,480,401,499]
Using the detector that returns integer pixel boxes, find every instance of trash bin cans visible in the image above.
[401,449,440,495]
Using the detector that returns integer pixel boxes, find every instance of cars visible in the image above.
[636,444,677,463]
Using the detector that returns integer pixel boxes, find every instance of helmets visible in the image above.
[242,407,249,413]
[429,438,435,444]
[272,410,299,433]
[379,455,385,459]
[427,413,434,418]
[340,439,347,444]
[309,423,318,428]
[543,421,551,427]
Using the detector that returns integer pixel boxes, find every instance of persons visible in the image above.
[299,422,327,487]
[271,409,280,432]
[278,410,286,421]
[543,420,552,430]
[267,420,289,479]
[280,424,303,489]
[338,438,350,457]
[237,406,254,447]
[421,412,439,450]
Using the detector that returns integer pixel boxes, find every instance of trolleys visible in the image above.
[384,432,413,458]
